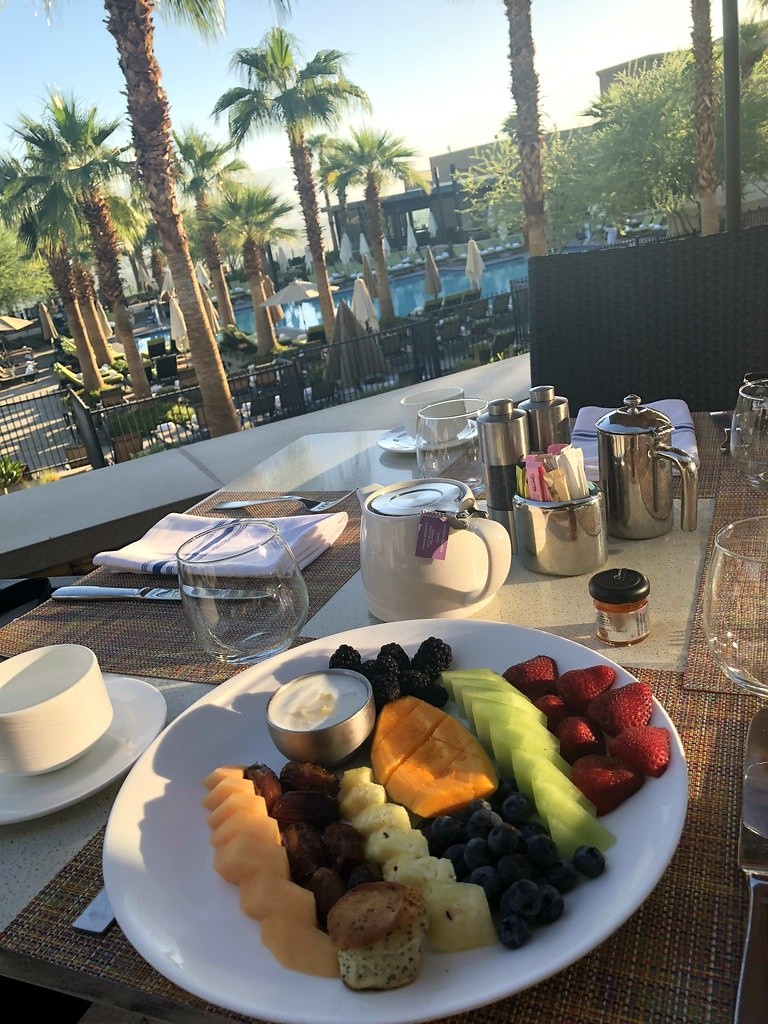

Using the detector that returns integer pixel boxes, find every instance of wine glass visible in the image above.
[701,516,767,838]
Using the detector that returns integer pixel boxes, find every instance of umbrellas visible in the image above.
[427,212,439,239]
[258,278,340,333]
[407,221,418,259]
[304,245,315,276]
[323,299,393,396]
[340,232,373,267]
[464,238,485,291]
[39,302,59,348]
[138,264,150,297]
[194,261,221,339]
[363,254,381,304]
[0,316,35,332]
[160,267,190,369]
[264,274,285,332]
[351,279,383,334]
[278,246,292,275]
[382,233,392,261]
[95,299,113,339]
[424,245,443,300]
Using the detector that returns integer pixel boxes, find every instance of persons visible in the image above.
[602,223,617,243]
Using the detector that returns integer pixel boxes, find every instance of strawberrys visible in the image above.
[502,654,670,814]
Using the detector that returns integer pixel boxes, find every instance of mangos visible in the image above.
[370,695,499,819]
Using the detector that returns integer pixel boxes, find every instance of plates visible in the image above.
[102,618,688,1024]
[0,679,167,827]
[377,419,478,453]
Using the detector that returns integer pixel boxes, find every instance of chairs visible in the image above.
[520,220,768,414]
[51,334,189,388]
[0,347,38,386]
[215,287,514,426]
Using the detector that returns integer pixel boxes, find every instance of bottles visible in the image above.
[476,398,532,556]
[517,385,572,453]
[587,569,650,646]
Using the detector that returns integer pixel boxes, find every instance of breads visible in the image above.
[328,878,430,993]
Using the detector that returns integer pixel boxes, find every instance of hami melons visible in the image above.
[204,764,344,976]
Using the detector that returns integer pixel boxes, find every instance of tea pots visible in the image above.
[356,478,512,622]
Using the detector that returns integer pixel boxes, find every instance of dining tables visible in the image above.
[0,413,767,1024]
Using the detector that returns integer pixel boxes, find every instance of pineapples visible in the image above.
[337,766,497,953]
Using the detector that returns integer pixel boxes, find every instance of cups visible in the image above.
[400,387,468,443]
[730,371,768,496]
[263,668,377,770]
[415,398,487,494]
[513,480,608,577]
[175,520,309,664]
[595,394,696,540]
[1,645,115,777]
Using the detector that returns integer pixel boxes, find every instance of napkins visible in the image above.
[568,395,706,484]
[93,509,349,579]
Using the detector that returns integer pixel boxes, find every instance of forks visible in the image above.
[213,489,359,512]
[707,408,751,451]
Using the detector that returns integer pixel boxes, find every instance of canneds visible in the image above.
[588,568,650,647]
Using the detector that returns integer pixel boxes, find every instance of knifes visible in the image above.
[733,706,767,1024]
[51,584,275,599]
